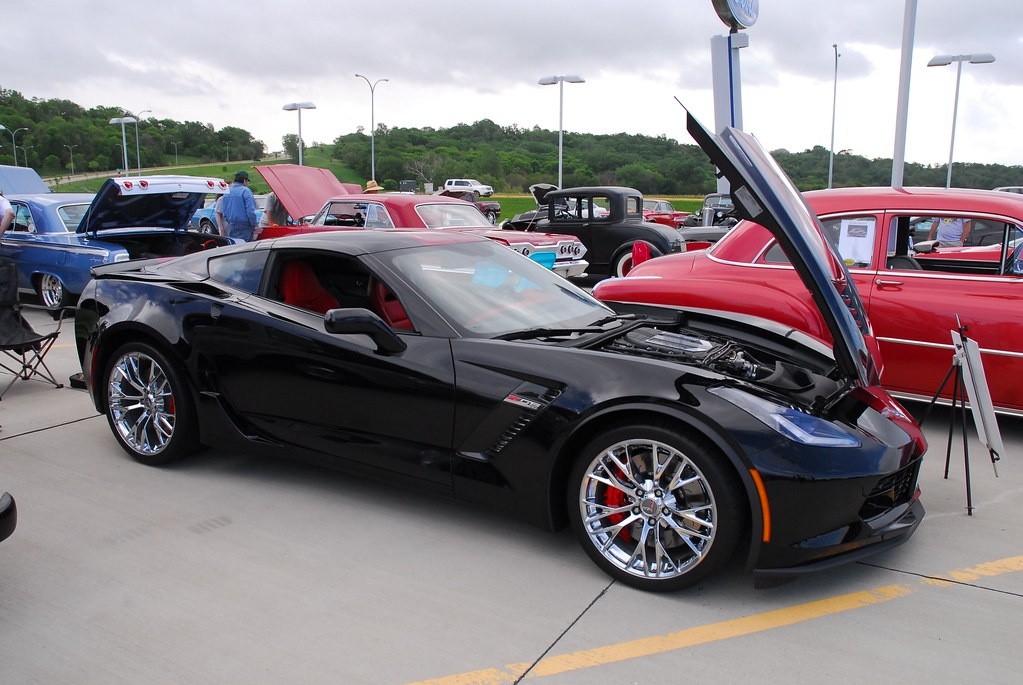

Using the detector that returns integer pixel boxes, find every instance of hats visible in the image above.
[363,180,384,193]
[235,170,250,181]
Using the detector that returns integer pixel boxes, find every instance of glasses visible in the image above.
[246,180,249,184]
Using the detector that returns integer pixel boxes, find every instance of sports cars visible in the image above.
[74,94,928,593]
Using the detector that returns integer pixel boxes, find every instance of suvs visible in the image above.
[445,179,494,197]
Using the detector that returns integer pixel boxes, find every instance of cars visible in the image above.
[418,189,501,224]
[598,197,691,229]
[501,184,686,277]
[592,185,1023,419]
[0,165,247,312]
[527,183,604,218]
[991,186,1023,194]
[253,164,592,326]
[907,216,1023,260]
[678,225,732,243]
[683,193,741,227]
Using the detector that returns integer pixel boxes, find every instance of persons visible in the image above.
[927,216,972,248]
[0,189,15,235]
[259,188,287,227]
[215,193,229,236]
[223,169,257,244]
[362,179,384,195]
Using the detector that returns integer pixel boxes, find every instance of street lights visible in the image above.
[64,144,78,175]
[120,108,151,176]
[538,75,586,189]
[170,142,181,165]
[0,124,34,168]
[926,53,997,188]
[108,117,137,176]
[829,43,842,188]
[354,73,390,181]
[223,141,232,161]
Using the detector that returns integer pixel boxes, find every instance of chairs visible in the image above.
[376,206,392,228]
[0,261,77,401]
[888,255,923,270]
[417,207,442,228]
[282,257,340,316]
[378,266,427,332]
